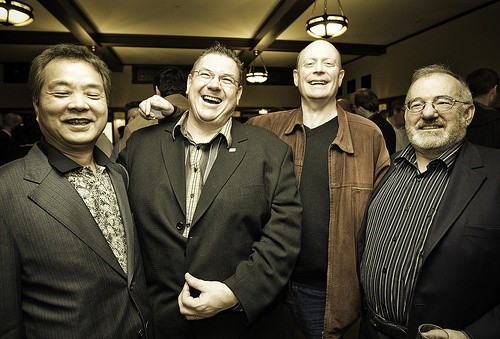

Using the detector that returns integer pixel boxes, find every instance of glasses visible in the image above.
[401,109,405,112]
[404,95,469,112]
[193,69,240,84]
[352,106,358,111]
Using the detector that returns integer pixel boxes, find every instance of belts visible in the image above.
[365,301,408,339]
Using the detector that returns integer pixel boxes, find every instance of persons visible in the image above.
[357,64,500,338]
[115,45,303,339]
[0,65,500,210]
[138,38,391,339]
[0,43,154,339]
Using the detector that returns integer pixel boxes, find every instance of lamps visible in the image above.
[246,50,270,83]
[306,0,349,39]
[0,0,34,28]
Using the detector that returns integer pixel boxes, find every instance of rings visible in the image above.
[194,316,197,321]
